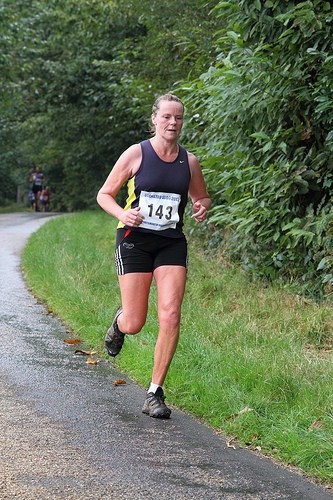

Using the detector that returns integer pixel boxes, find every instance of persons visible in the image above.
[28,190,36,211]
[29,166,45,213]
[97,93,212,420]
[40,186,50,212]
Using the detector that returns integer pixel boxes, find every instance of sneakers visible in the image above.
[105,306,126,357]
[143,386,171,419]
